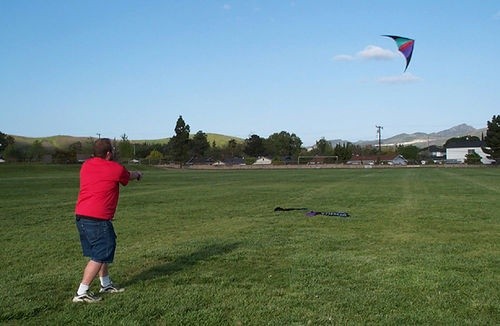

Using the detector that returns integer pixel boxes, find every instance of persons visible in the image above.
[71,138,141,304]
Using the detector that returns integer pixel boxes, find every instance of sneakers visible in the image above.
[99,284,125,293]
[72,291,103,304]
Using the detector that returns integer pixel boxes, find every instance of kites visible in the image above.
[380,34,415,72]
